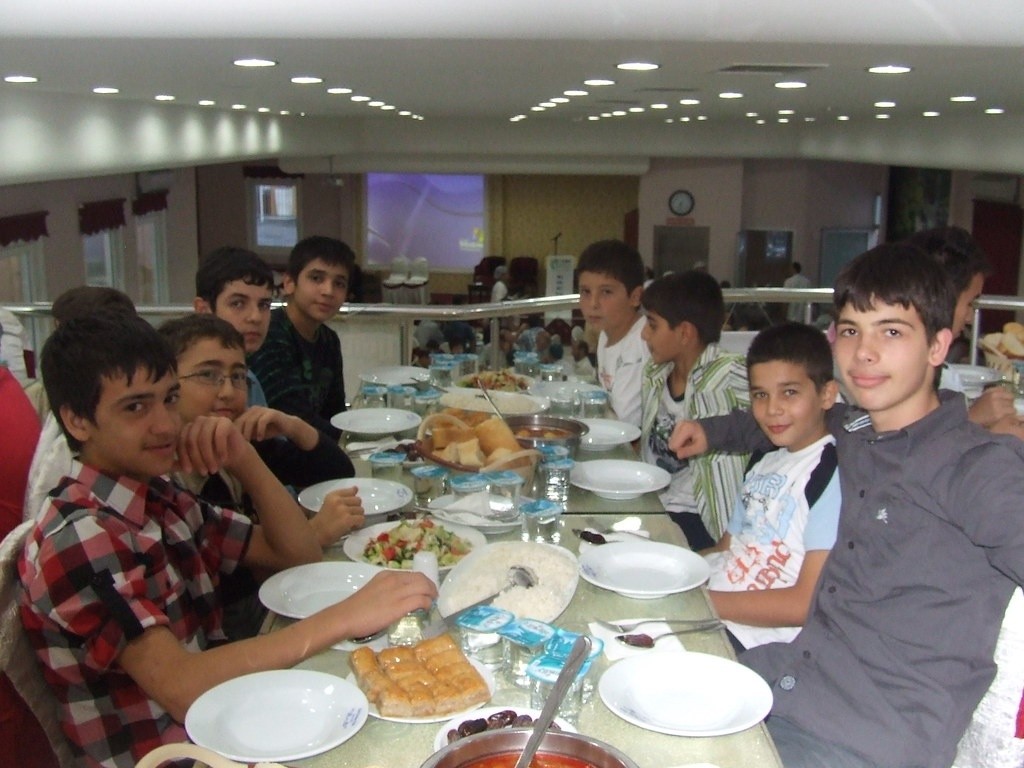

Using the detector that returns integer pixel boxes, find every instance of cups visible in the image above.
[454,604,604,726]
[368,446,575,546]
[387,609,428,648]
[360,351,608,415]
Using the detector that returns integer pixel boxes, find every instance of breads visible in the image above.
[427,415,527,468]
[351,634,489,717]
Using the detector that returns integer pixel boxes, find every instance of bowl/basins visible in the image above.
[419,727,639,768]
[502,415,589,457]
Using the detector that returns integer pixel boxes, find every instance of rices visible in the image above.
[446,537,577,622]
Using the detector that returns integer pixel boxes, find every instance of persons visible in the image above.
[1,233,367,549]
[696,322,840,654]
[909,225,1024,440]
[637,266,752,552]
[12,308,436,767]
[414,258,811,374]
[737,238,1024,768]
[577,236,649,456]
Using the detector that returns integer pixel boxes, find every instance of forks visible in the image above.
[594,616,719,632]
[585,517,650,541]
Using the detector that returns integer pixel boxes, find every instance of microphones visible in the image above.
[553,232,562,240]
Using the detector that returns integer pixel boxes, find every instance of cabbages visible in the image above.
[459,369,529,391]
[364,519,470,568]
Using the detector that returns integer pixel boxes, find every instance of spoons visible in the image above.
[442,565,539,625]
[614,622,726,648]
[571,528,610,545]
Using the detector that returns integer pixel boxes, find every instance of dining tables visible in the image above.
[193,375,785,768]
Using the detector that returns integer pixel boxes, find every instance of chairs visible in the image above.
[0,377,88,768]
[952,584,1024,767]
[466,256,539,304]
[381,255,431,306]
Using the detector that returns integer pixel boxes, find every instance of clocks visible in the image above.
[669,189,694,216]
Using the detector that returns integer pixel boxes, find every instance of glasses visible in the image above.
[177,368,255,391]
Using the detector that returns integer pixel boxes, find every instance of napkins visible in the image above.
[577,525,650,553]
[587,618,686,663]
[433,491,515,524]
[345,435,425,465]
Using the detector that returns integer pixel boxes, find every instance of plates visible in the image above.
[569,459,671,500]
[434,541,578,625]
[297,477,413,516]
[356,365,429,386]
[185,669,369,762]
[578,541,711,599]
[577,418,641,450]
[438,387,550,416]
[429,494,535,534]
[258,560,384,619]
[329,407,422,440]
[344,657,497,723]
[598,649,774,737]
[343,520,487,572]
